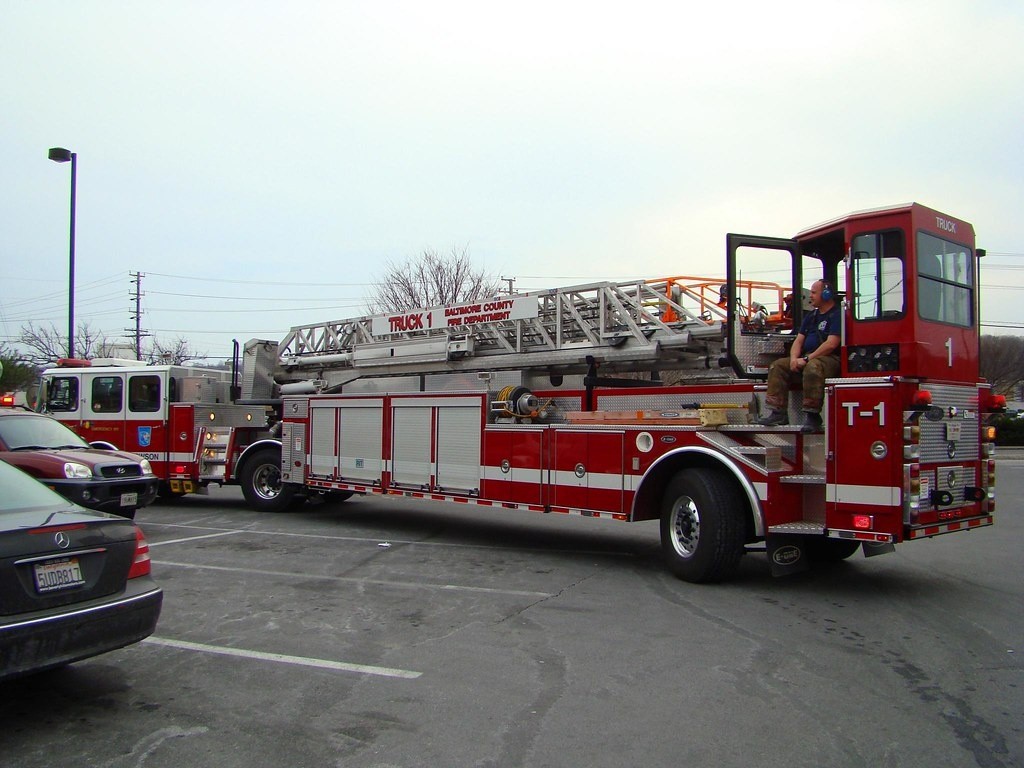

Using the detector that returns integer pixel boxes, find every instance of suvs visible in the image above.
[0,396,160,521]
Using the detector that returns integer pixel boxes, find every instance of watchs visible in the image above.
[803,356,809,364]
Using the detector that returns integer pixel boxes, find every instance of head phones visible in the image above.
[818,279,832,301]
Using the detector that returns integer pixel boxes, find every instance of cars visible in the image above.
[0,459,165,679]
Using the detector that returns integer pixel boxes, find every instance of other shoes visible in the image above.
[800,413,824,434]
[757,409,790,426]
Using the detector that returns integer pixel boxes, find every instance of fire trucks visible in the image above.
[31,201,1008,586]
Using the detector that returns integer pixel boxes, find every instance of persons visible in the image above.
[715,285,751,325]
[757,279,841,433]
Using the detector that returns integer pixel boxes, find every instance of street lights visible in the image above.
[48,146,77,363]
